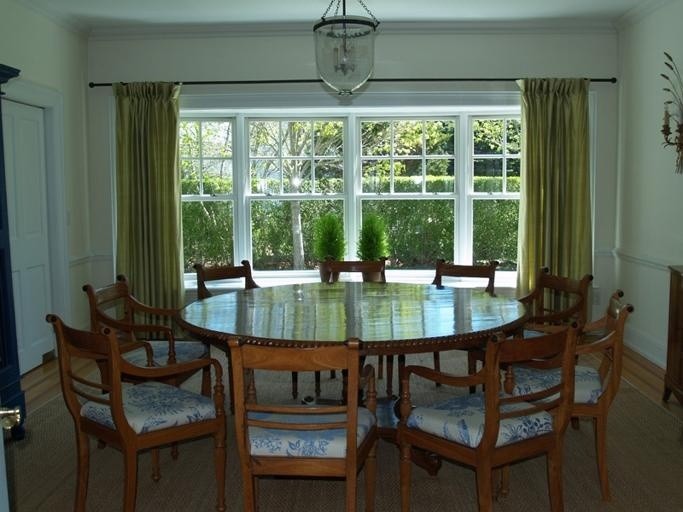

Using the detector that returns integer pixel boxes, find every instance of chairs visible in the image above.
[228,337,377,511]
[83,276,212,406]
[46,314,228,511]
[397,321,577,512]
[193,260,265,399]
[486,289,635,499]
[402,259,498,395]
[291,256,388,401]
[469,267,594,428]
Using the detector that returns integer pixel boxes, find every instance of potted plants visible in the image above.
[359,211,390,281]
[313,211,345,282]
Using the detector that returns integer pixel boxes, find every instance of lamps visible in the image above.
[313,0,382,96]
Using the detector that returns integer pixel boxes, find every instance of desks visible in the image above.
[661,265,683,407]
[176,282,527,476]
